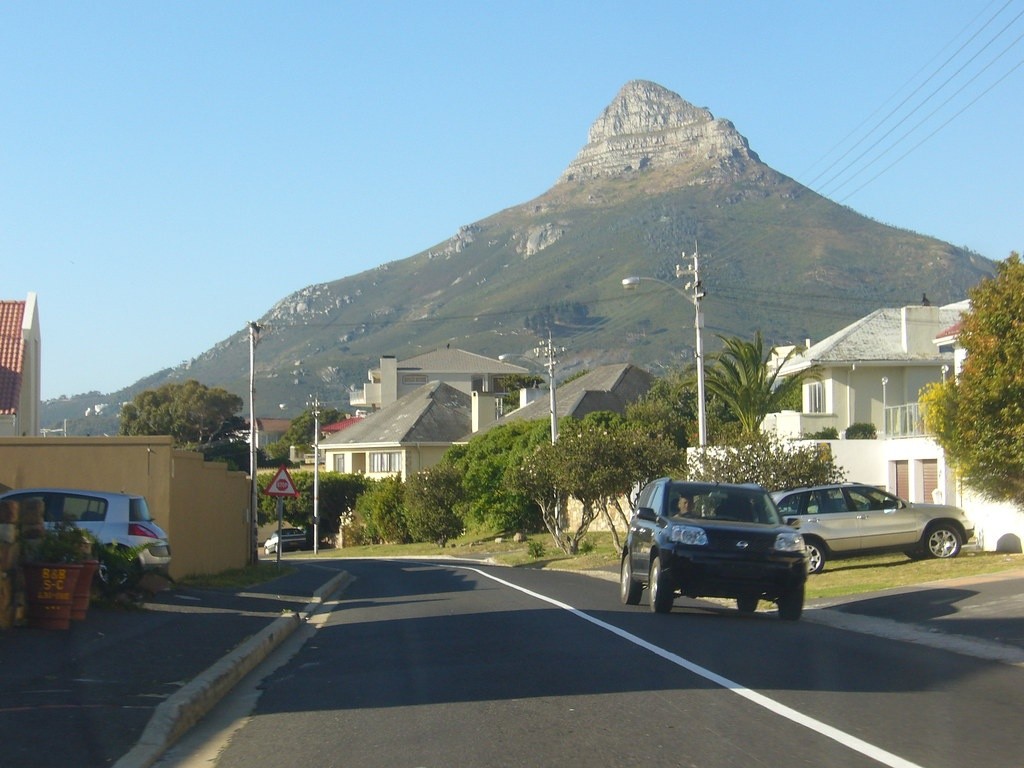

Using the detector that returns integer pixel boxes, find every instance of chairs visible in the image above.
[81,511,102,521]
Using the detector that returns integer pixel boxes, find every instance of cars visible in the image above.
[263,527,306,555]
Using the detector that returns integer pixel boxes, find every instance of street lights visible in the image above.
[499,353,562,547]
[621,276,707,481]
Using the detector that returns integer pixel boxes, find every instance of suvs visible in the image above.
[620,477,808,620]
[0,487,172,591]
[769,482,975,574]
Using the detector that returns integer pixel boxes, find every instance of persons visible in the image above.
[0,498,47,632]
[671,494,704,519]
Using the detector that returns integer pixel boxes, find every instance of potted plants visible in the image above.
[16,515,99,631]
